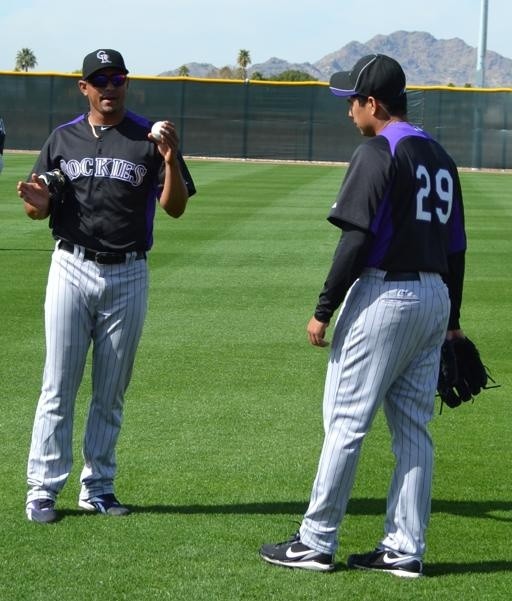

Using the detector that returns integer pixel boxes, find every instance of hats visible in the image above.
[330,53,406,98]
[83,49,129,78]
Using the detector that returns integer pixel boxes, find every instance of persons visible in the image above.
[256,52,494,579]
[16,48,198,522]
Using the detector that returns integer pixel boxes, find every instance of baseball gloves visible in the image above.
[435,337,486,408]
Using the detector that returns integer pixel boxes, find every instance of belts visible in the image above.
[384,272,420,282]
[58,240,143,265]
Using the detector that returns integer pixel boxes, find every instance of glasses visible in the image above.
[85,74,127,88]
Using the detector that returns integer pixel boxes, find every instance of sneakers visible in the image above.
[260,535,332,569]
[347,548,424,578]
[25,499,59,524]
[78,492,129,517]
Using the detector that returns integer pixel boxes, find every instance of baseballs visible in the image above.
[151,121,167,141]
